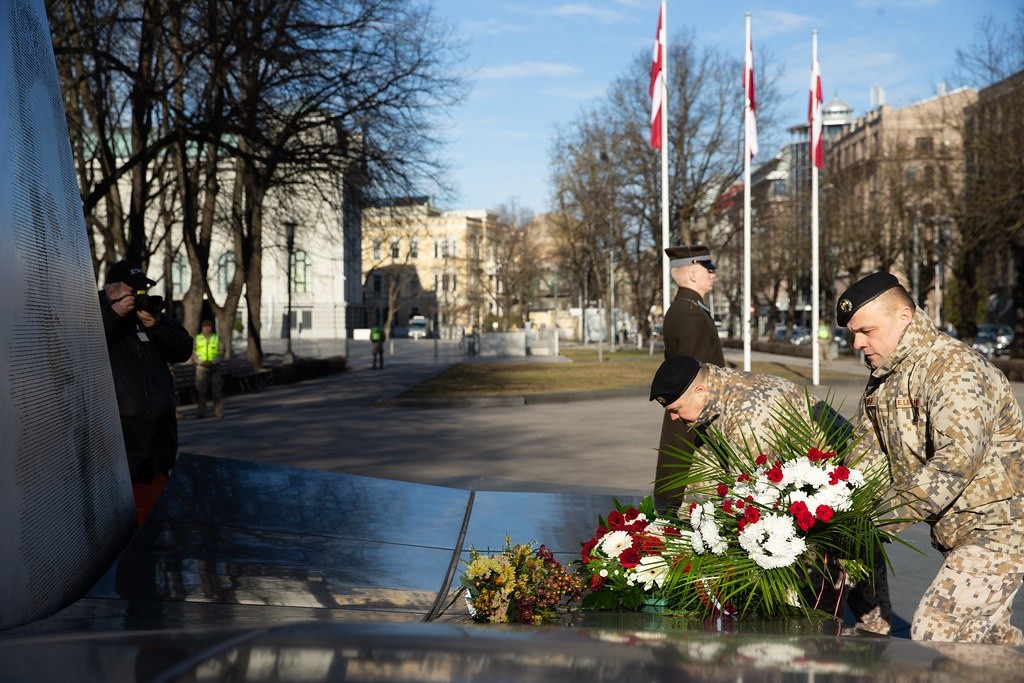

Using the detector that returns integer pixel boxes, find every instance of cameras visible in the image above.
[132,294,164,313]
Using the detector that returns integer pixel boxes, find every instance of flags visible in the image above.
[744,35,759,162]
[808,52,825,168]
[649,3,662,151]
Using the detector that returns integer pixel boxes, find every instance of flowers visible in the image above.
[460,537,575,626]
[577,445,867,594]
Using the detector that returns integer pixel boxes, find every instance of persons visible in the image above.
[649,354,893,636]
[370,322,386,370]
[837,273,1024,644]
[96,260,193,529]
[193,321,224,419]
[654,247,725,495]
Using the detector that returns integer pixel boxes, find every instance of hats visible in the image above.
[836,271,898,327]
[664,244,716,270]
[104,259,157,288]
[649,353,699,408]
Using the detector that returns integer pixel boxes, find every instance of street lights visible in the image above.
[280,222,298,365]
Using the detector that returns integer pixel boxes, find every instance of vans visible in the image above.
[408,316,430,339]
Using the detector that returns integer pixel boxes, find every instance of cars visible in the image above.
[715,318,849,350]
[937,324,1015,360]
